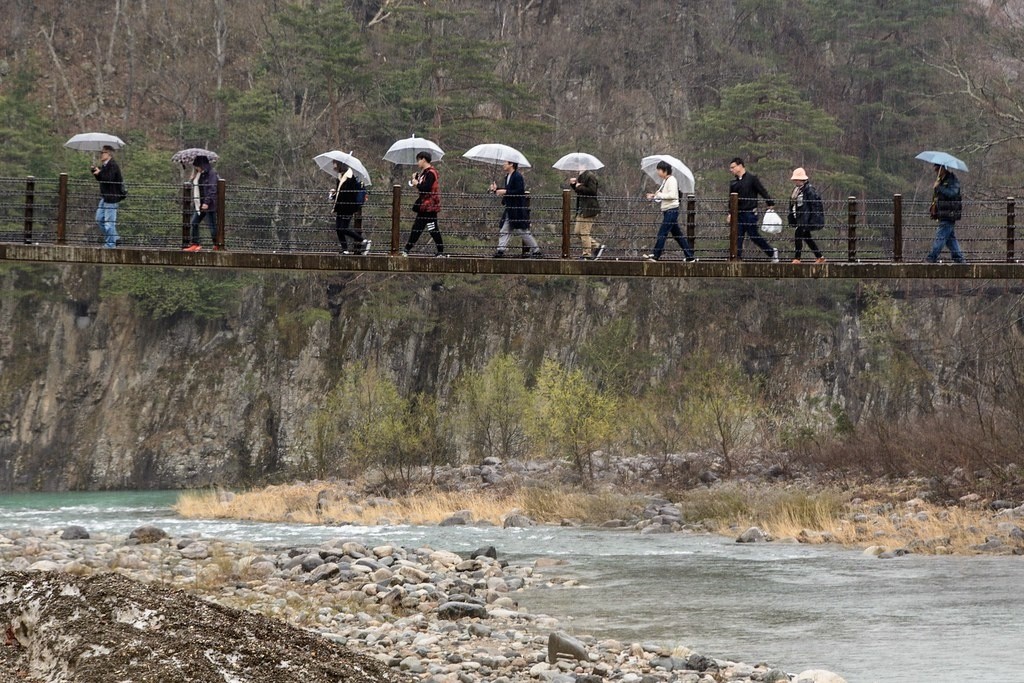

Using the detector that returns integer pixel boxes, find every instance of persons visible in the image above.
[89,144,128,249]
[181,156,221,252]
[569,157,606,260]
[725,157,780,263]
[329,158,371,256]
[401,153,449,258]
[644,161,699,264]
[489,160,543,258]
[787,167,827,264]
[921,163,968,263]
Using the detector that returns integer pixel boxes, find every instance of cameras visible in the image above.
[92,166,100,173]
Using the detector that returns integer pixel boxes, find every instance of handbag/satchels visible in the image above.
[761,209,782,234]
[930,202,938,220]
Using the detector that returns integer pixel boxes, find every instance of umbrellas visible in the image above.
[63,132,126,166]
[551,152,605,174]
[381,133,445,188]
[311,150,372,200]
[171,147,220,168]
[914,151,970,173]
[640,154,696,203]
[461,142,532,185]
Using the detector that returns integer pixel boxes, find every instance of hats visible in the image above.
[791,167,808,181]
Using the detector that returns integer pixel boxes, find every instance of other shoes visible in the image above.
[340,249,350,255]
[643,254,658,262]
[399,251,409,257]
[814,256,826,263]
[683,257,699,262]
[522,251,543,259]
[592,244,606,260]
[791,259,802,264]
[212,246,220,251]
[770,248,779,263]
[493,251,505,256]
[574,256,593,260]
[433,253,449,258]
[361,239,372,255]
[182,244,201,252]
[115,240,122,246]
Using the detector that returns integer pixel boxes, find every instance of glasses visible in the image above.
[100,151,108,154]
